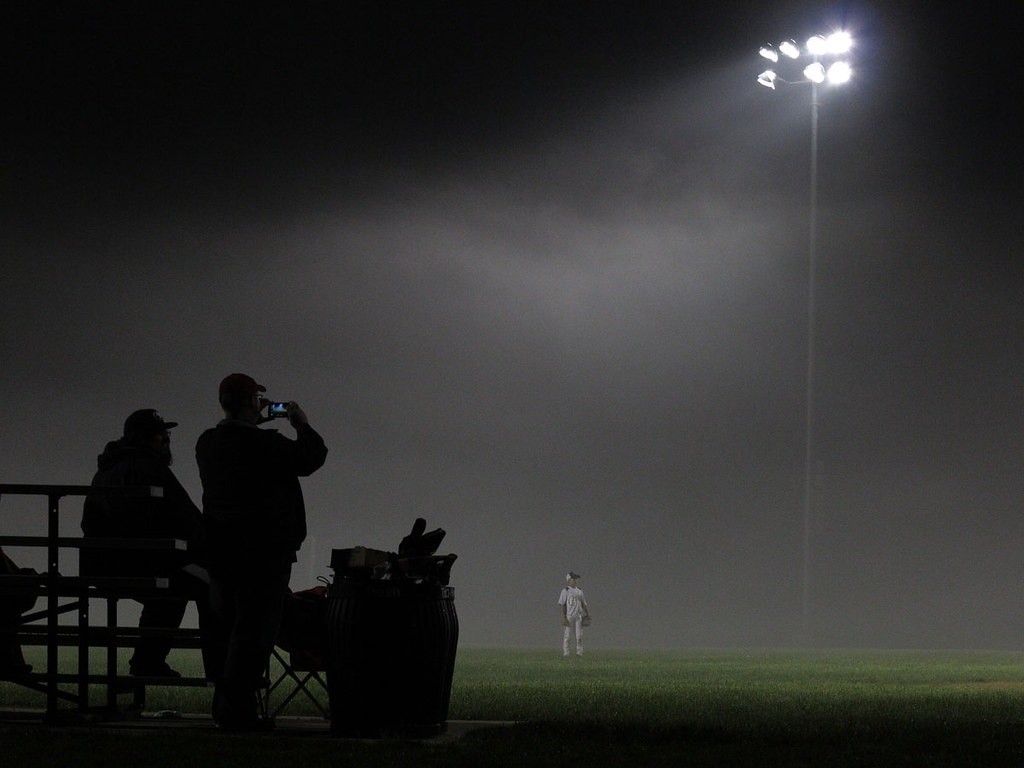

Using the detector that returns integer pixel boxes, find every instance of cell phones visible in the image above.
[268,402,290,417]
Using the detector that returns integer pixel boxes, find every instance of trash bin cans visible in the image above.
[319,579,459,737]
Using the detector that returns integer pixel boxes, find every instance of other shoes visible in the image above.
[564,653,571,656]
[260,675,274,689]
[128,658,184,681]
[0,663,35,680]
[577,651,584,657]
[212,712,279,732]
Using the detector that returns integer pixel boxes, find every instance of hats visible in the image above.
[217,372,268,403]
[566,571,580,581]
[123,407,179,440]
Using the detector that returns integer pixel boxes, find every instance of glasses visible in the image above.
[253,394,264,400]
[155,429,173,438]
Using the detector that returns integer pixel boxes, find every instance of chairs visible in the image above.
[255,591,330,723]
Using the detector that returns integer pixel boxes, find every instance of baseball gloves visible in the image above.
[581,615,592,627]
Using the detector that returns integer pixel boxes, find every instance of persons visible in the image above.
[196,374,328,733]
[77,409,204,679]
[559,572,592,656]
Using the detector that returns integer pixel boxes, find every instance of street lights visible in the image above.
[756,28,861,650]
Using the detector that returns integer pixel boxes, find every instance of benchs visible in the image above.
[0,475,296,732]
